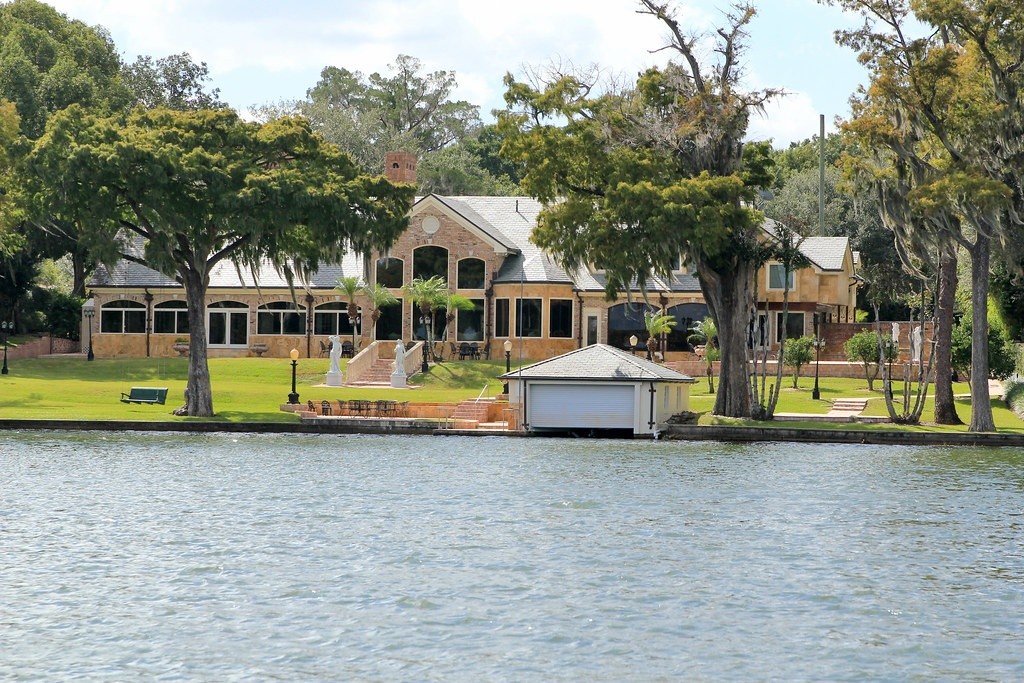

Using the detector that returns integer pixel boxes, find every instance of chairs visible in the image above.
[318,335,491,360]
[307,398,409,419]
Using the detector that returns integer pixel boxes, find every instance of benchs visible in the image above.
[119,386,168,405]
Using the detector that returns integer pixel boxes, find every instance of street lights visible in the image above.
[2,318,13,372]
[286,350,300,404]
[629,334,638,357]
[501,340,514,395]
[84,306,96,362]
[349,317,361,359]
[419,315,431,372]
